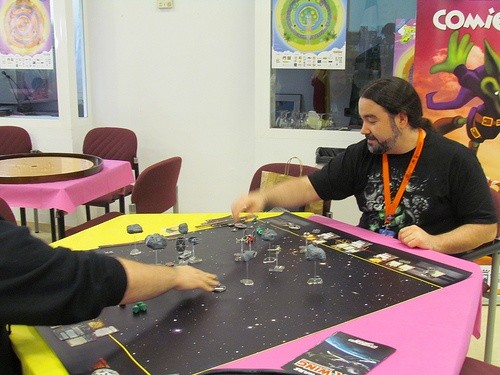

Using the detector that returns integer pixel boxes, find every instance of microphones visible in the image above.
[2,71,33,113]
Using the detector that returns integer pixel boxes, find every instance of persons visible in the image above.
[0,218,220,375]
[348,23,395,126]
[310,69,329,114]
[231,77,498,258]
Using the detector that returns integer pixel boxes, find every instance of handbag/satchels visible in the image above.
[260,157,323,216]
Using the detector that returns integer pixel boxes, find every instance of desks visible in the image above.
[0,160,133,243]
[6,212,483,374]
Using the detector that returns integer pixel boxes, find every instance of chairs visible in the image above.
[0,125,182,237]
[250,162,333,219]
[452,184,500,364]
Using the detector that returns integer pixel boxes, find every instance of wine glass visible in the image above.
[275,109,335,129]
